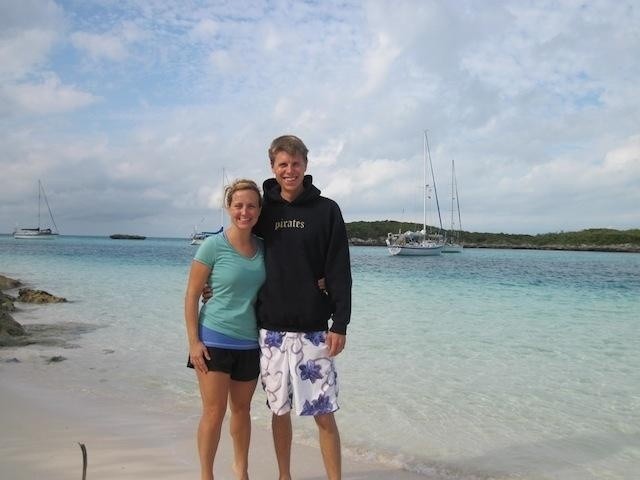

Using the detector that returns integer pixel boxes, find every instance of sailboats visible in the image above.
[190,167,230,245]
[13,179,60,239]
[385,129,463,256]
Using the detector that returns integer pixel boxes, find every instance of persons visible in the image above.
[202,135,352,480]
[185,179,325,480]
